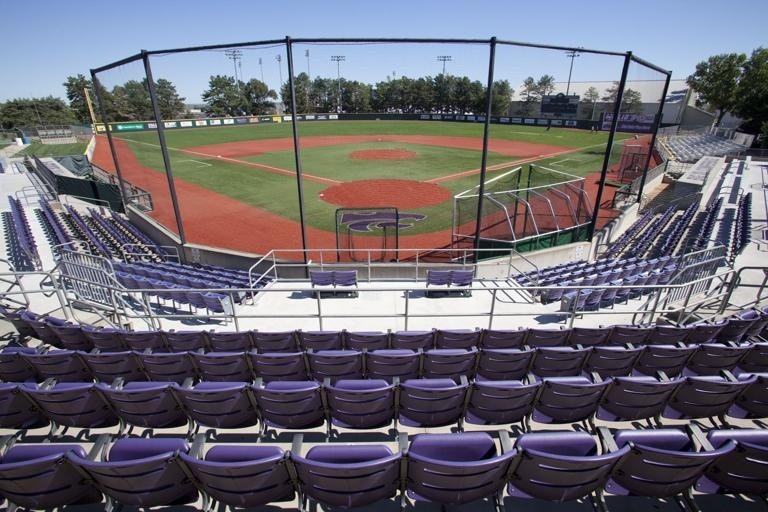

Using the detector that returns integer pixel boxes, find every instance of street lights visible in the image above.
[330,54,345,90]
[564,46,584,96]
[275,54,283,87]
[237,61,243,82]
[258,58,264,82]
[225,47,240,84]
[435,54,451,78]
[303,47,310,78]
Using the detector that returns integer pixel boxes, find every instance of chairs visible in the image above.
[425,270,474,297]
[510,192,753,324]
[309,270,359,296]
[7,193,276,326]
[663,132,749,161]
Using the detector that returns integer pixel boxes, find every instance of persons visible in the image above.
[596,125,598,133]
[591,125,594,131]
[634,134,638,139]
